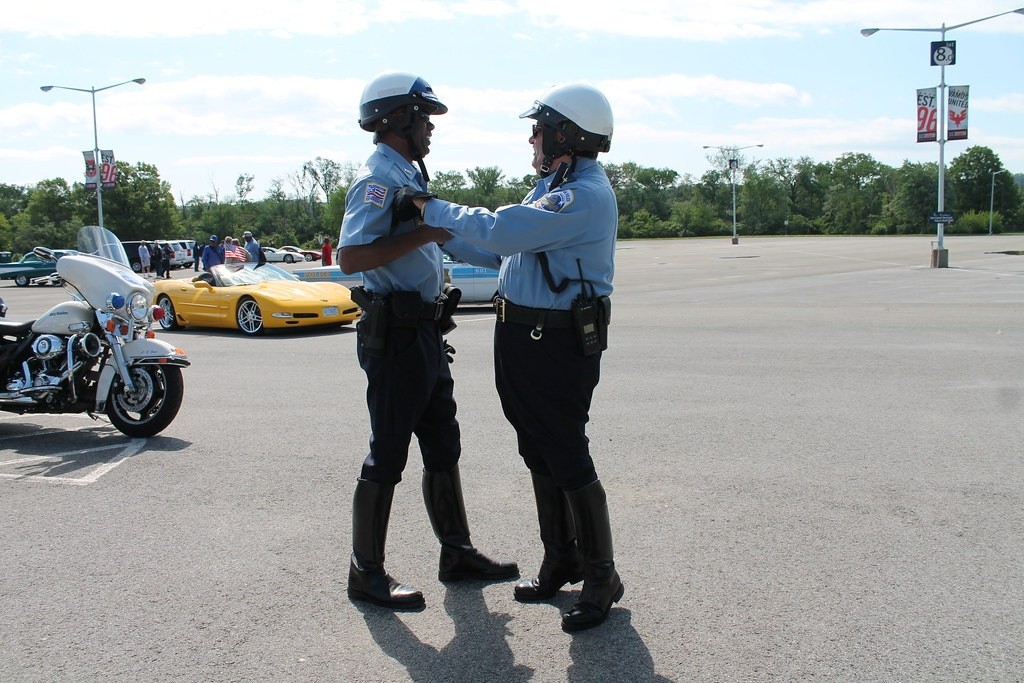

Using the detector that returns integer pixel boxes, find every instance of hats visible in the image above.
[210,235,218,242]
[241,231,253,238]
[323,237,330,242]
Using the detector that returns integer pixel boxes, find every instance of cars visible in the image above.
[0,250,78,286]
[293,248,500,303]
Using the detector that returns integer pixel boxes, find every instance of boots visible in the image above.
[561,478,625,630]
[422,463,520,583]
[348,477,424,609]
[514,469,588,602]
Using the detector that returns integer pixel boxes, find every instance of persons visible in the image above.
[192,231,260,272]
[138,240,173,280]
[322,237,332,265]
[392,83,626,634]
[336,72,519,611]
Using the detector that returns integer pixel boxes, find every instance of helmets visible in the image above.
[357,71,449,132]
[519,83,614,153]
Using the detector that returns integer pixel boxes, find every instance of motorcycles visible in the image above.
[0,225,190,437]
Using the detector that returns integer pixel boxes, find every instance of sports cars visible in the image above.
[261,247,304,264]
[278,246,322,262]
[150,260,361,336]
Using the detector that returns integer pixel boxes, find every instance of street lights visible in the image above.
[40,77,147,228]
[860,8,1024,268]
[703,145,764,244]
[989,169,1006,235]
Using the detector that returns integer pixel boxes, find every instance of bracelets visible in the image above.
[421,200,427,219]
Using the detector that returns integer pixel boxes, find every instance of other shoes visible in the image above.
[143,273,173,280]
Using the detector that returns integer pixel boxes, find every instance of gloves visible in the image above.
[392,184,436,223]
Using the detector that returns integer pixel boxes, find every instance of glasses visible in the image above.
[413,110,431,124]
[532,123,544,138]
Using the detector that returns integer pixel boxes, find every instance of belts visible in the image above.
[492,295,573,328]
[376,295,444,321]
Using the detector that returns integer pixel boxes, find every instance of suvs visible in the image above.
[178,240,199,268]
[156,240,186,268]
[88,241,161,273]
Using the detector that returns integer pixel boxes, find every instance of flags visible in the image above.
[225,243,246,262]
